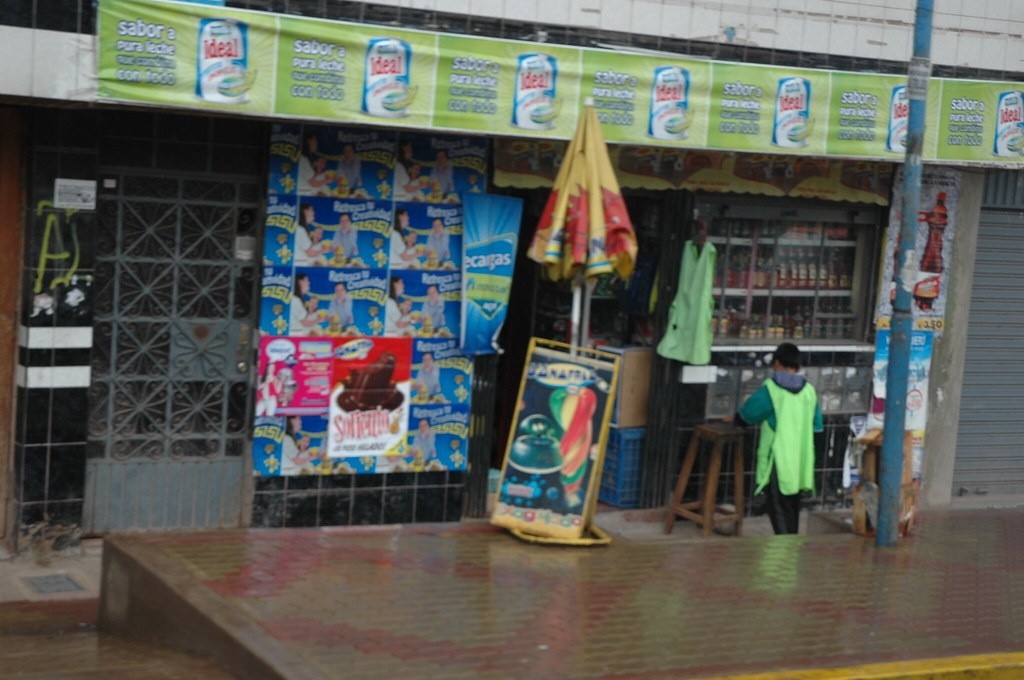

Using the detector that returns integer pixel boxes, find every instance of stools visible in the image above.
[664,423,747,544]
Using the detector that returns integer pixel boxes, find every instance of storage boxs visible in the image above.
[595,346,653,510]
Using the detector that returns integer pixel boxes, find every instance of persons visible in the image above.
[249,118,472,472]
[735,341,819,536]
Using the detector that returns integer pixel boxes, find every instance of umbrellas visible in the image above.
[527,96,637,354]
